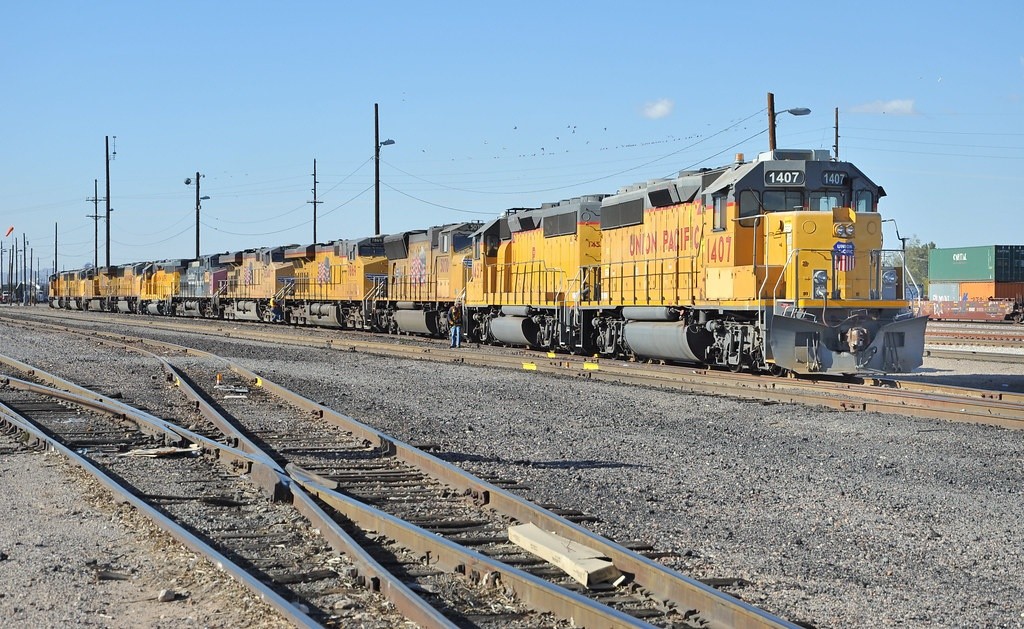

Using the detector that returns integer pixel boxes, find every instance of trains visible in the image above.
[49,148,929,388]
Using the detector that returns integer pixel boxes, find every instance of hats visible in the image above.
[454,302,461,306]
[272,294,275,297]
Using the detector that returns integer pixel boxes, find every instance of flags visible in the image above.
[5,225,14,237]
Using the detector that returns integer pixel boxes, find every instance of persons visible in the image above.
[447,302,461,349]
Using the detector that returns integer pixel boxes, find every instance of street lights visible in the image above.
[185,171,210,262]
[767,91,811,154]
[374,102,395,238]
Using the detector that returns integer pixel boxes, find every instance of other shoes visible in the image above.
[450,344,456,348]
[271,318,277,324]
[457,345,460,348]
